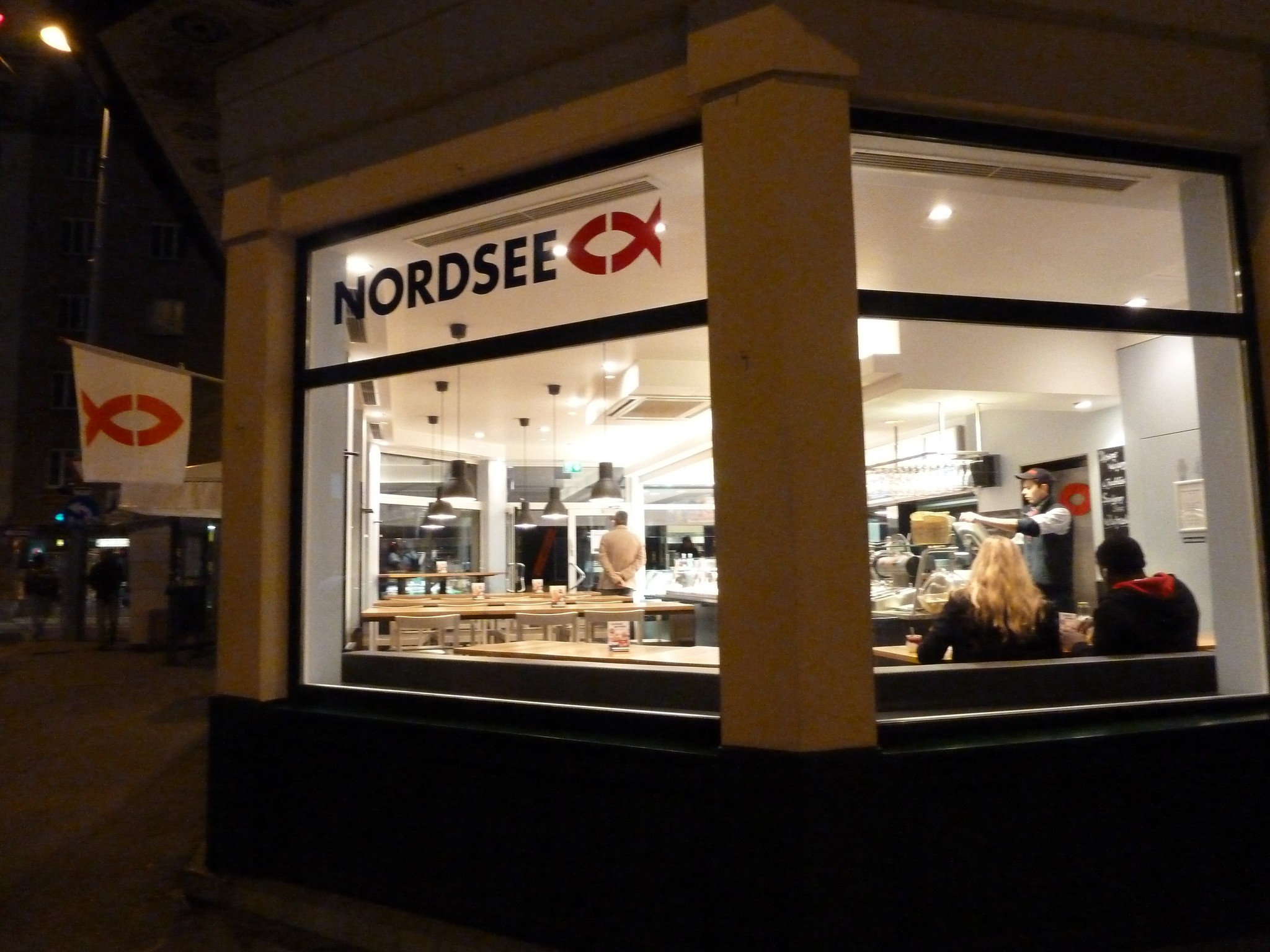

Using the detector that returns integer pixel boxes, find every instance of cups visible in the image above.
[905,635,920,653]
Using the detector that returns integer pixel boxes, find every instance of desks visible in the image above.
[873,620,1093,663]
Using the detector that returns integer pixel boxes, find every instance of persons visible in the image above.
[915,535,1064,664]
[959,467,1078,615]
[679,535,700,560]
[23,552,59,639]
[596,511,646,640]
[1057,535,1200,658]
[88,546,130,648]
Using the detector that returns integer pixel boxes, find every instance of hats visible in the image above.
[1013,467,1059,486]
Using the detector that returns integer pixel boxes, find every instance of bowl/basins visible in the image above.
[934,558,951,570]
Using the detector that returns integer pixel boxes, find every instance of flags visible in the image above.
[71,345,193,485]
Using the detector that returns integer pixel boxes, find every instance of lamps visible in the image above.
[419,323,625,534]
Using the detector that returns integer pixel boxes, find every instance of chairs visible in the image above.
[359,589,698,649]
